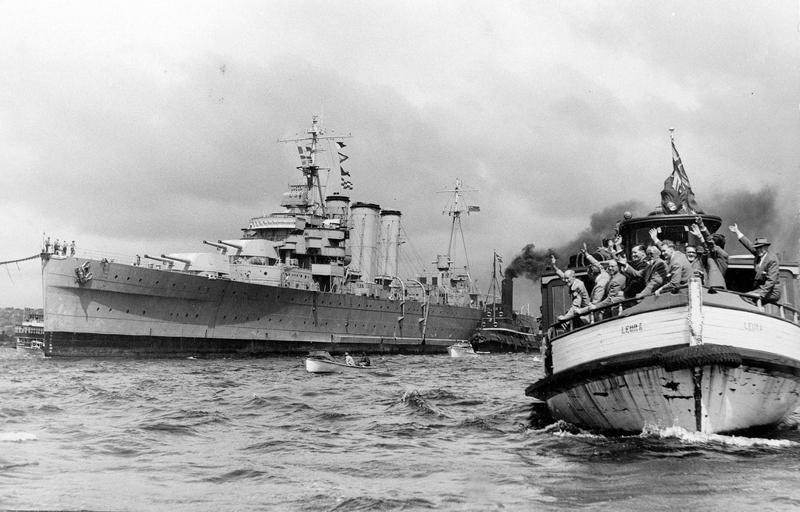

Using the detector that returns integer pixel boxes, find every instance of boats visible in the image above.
[14,307,44,353]
[446,342,474,358]
[306,351,378,376]
[523,208,799,438]
[470,279,540,353]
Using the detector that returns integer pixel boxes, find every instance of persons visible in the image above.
[548,215,733,328]
[727,222,783,306]
[53,239,61,255]
[135,253,141,266]
[44,236,53,252]
[355,350,370,367]
[343,352,356,366]
[70,240,75,256]
[61,240,69,256]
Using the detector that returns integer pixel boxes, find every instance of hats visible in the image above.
[751,237,771,248]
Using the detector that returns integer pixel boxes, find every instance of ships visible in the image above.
[39,115,485,361]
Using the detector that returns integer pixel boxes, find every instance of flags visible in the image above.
[468,205,481,212]
[340,165,350,176]
[661,144,708,216]
[340,176,354,191]
[337,152,349,164]
[334,140,347,150]
